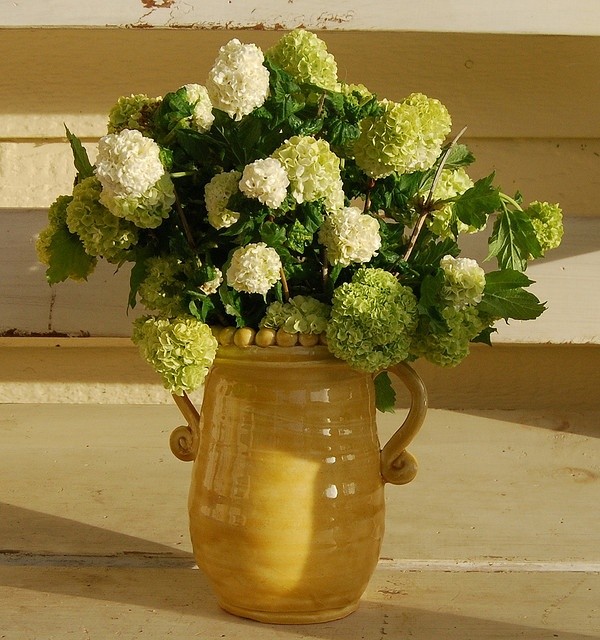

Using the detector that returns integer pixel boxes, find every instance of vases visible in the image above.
[166,342,429,626]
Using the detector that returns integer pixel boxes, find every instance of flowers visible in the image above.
[31,29,565,398]
